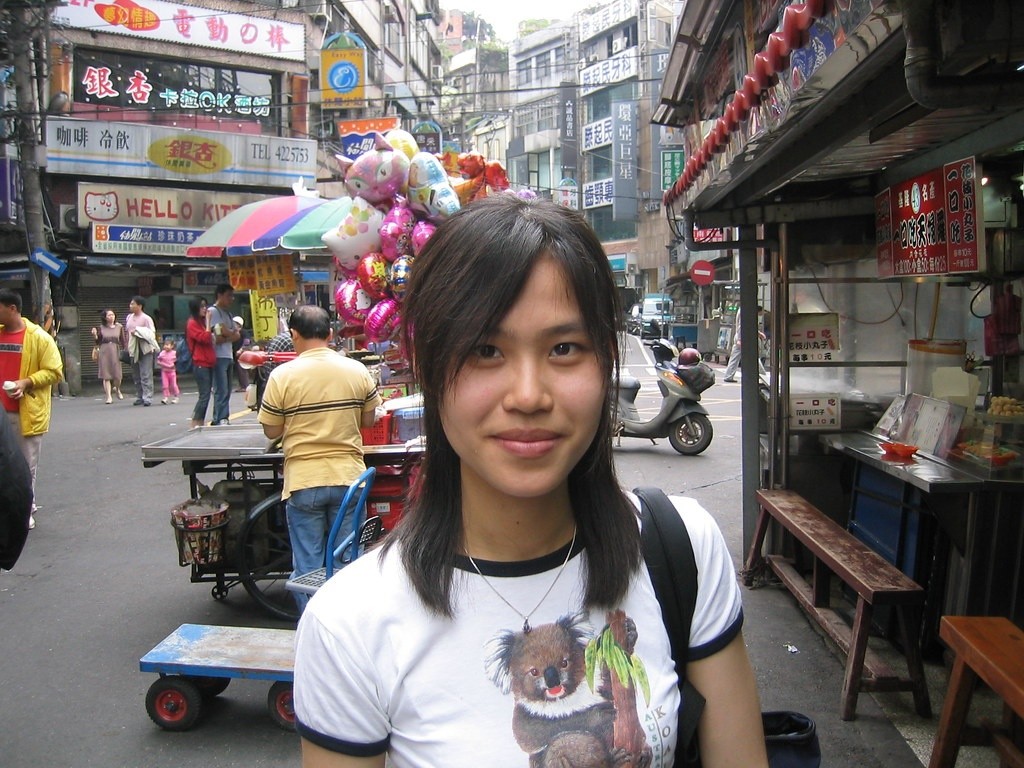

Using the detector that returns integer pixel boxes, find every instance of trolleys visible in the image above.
[696,280,768,367]
[141,425,426,623]
[140,466,377,733]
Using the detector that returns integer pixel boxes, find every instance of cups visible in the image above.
[3,383,16,398]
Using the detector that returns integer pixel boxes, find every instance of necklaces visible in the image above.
[465,534,576,634]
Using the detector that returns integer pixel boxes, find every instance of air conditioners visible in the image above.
[432,65,443,78]
[612,36,627,53]
[386,5,396,15]
[60,203,87,234]
[578,58,587,70]
[302,0,333,22]
[590,54,599,61]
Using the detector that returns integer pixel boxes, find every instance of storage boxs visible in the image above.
[394,407,429,442]
[360,413,390,446]
[366,463,424,532]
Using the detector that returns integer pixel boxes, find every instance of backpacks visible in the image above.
[175,315,198,374]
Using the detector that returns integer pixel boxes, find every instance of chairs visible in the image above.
[285,515,381,595]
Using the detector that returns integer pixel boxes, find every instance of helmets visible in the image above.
[678,347,702,366]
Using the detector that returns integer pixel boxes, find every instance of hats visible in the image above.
[232,315,244,326]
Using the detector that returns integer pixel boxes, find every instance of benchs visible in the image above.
[744,490,930,722]
[931,615,1024,768]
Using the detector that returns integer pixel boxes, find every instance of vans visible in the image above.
[639,293,672,338]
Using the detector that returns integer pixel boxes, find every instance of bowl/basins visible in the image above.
[893,446,919,457]
[880,443,904,455]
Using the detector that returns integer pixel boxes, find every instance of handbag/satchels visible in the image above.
[632,484,823,768]
[91,327,100,362]
[120,350,131,364]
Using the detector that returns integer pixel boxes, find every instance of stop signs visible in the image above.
[690,260,715,285]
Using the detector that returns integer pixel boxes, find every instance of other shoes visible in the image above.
[161,397,168,405]
[113,387,124,400]
[28,504,37,529]
[105,396,113,404]
[172,397,180,403]
[143,401,151,406]
[133,398,144,405]
[724,379,737,382]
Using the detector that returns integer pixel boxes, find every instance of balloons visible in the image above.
[321,129,537,373]
[187,196,352,305]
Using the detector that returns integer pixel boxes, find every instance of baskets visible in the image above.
[360,410,392,445]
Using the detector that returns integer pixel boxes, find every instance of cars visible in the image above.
[626,302,643,335]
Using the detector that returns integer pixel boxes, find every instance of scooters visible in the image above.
[610,318,715,455]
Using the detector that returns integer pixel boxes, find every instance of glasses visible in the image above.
[200,304,210,307]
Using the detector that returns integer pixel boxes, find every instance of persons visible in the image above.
[0,286,63,571]
[187,283,295,429]
[725,307,771,383]
[92,297,179,407]
[257,305,382,615]
[294,195,768,768]
